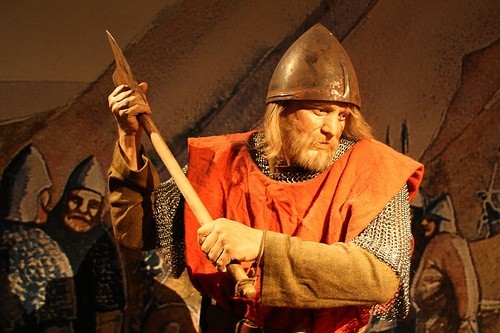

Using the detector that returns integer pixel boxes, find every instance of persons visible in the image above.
[108,23,424,333]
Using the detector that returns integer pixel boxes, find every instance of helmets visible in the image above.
[265,23,362,110]
